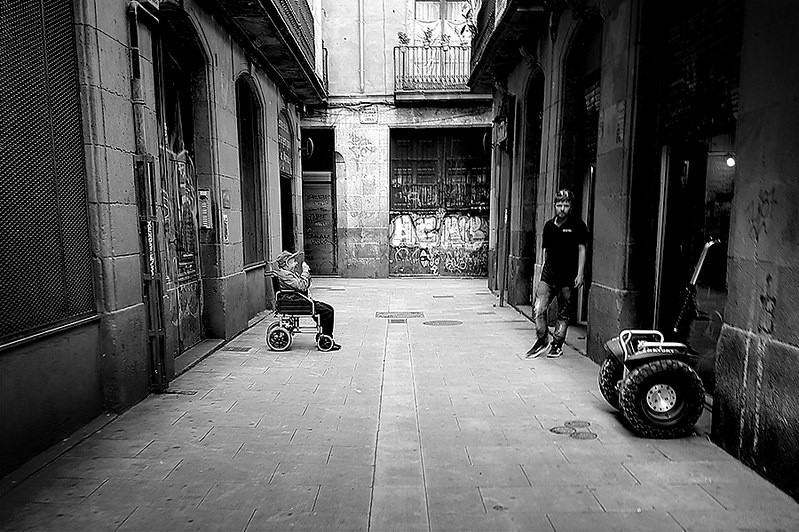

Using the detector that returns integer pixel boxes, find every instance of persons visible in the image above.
[276,251,341,350]
[525,190,590,359]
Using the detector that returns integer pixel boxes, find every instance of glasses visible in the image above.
[557,190,573,199]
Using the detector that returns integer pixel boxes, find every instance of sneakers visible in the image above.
[547,341,563,357]
[526,340,551,358]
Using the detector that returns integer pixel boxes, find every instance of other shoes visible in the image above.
[319,342,341,351]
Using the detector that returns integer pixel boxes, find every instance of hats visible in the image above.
[276,250,299,267]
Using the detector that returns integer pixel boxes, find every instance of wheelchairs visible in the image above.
[264,270,334,352]
